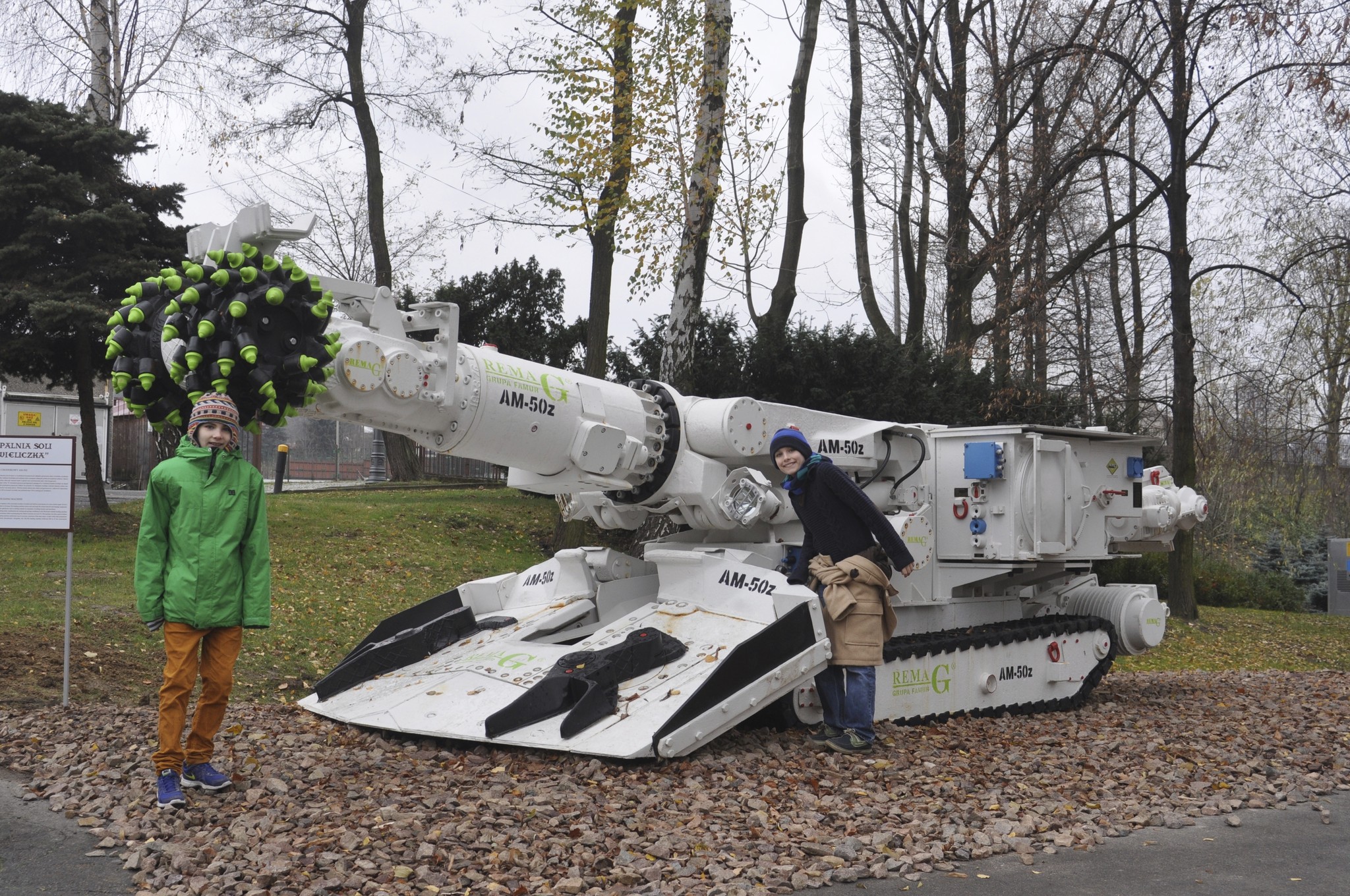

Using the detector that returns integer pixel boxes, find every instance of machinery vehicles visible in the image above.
[105,245,1207,758]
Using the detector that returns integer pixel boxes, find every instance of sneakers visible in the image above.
[180,761,232,791]
[156,768,188,808]
[808,727,845,746]
[824,731,871,754]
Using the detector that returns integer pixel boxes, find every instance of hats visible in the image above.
[770,428,813,470]
[186,392,239,452]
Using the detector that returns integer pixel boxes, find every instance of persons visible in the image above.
[768,428,917,755]
[131,389,271,809]
